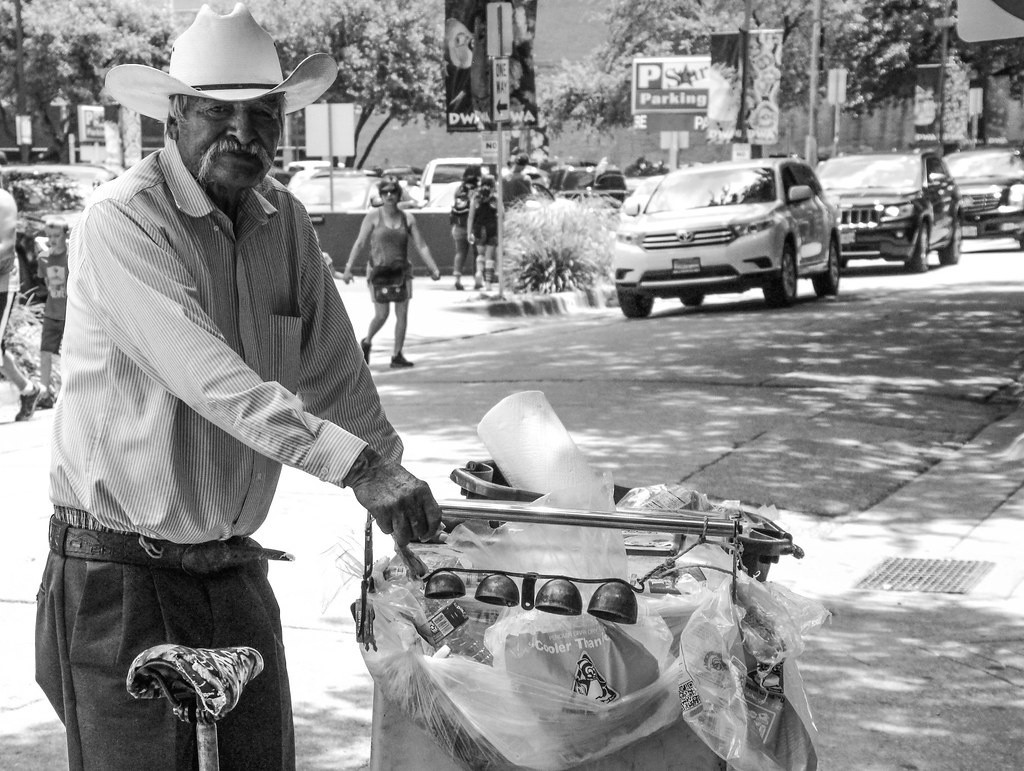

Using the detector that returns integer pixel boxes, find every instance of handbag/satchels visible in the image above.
[15,223,50,303]
[367,264,408,303]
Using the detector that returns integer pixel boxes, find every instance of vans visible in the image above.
[419,157,481,209]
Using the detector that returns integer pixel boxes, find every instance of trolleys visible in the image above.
[359,502,745,645]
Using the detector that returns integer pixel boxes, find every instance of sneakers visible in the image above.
[15,382,42,421]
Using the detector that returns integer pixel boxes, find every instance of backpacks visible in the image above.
[449,182,479,228]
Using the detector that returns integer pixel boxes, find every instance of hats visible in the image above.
[515,152,529,166]
[104,3,338,123]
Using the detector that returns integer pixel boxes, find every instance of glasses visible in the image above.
[378,188,397,195]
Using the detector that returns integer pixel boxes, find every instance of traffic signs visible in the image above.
[493,57,512,123]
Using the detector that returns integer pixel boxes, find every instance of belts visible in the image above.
[47,513,296,577]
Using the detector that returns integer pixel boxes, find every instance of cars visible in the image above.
[273,166,423,208]
[1,162,117,223]
[945,148,1023,249]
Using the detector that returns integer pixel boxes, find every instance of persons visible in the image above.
[449,163,499,291]
[34,4,440,771]
[502,154,533,212]
[36,217,68,409]
[0,188,43,421]
[342,175,440,367]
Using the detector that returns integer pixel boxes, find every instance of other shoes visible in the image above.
[390,351,414,368]
[360,337,372,364]
[35,397,53,408]
[455,282,464,290]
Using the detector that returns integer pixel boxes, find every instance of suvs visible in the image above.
[612,154,845,317]
[817,152,964,273]
